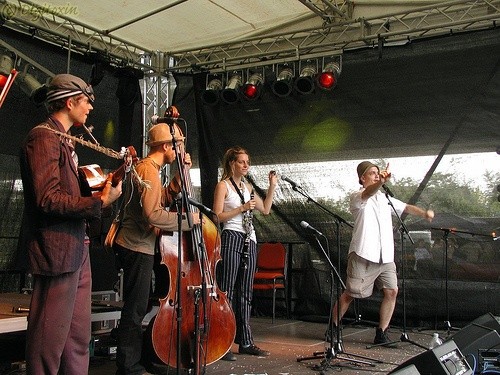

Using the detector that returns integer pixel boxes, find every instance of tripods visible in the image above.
[292,186,500,375]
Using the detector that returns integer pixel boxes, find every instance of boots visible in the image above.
[325,323,342,343]
[373,327,398,347]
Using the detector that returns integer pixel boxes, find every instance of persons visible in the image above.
[324,161,435,348]
[213,145,278,361]
[13,74,123,375]
[110,123,201,375]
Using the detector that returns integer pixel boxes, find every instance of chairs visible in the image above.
[253,243,290,324]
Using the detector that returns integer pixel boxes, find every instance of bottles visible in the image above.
[26,274,33,289]
[428,332,442,350]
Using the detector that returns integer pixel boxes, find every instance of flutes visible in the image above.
[239,188,255,270]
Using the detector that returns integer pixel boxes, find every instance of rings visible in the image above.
[107,181,112,184]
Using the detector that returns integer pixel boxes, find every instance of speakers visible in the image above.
[387,312,500,375]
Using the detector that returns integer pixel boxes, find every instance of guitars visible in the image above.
[78,146,139,196]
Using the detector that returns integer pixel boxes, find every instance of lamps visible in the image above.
[204,73,224,106]
[271,62,296,97]
[242,65,264,102]
[88,54,111,87]
[222,69,244,104]
[295,58,318,94]
[317,55,342,91]
[116,67,136,98]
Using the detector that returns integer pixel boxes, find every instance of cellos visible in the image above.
[140,106,237,375]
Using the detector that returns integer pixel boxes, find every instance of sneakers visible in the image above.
[221,351,237,361]
[238,344,271,356]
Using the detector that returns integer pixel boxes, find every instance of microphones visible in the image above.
[281,175,301,189]
[382,184,394,197]
[300,221,326,238]
[178,192,216,215]
[151,115,184,125]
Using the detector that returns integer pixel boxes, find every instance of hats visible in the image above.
[46,74,94,102]
[146,123,185,145]
[357,161,379,185]
